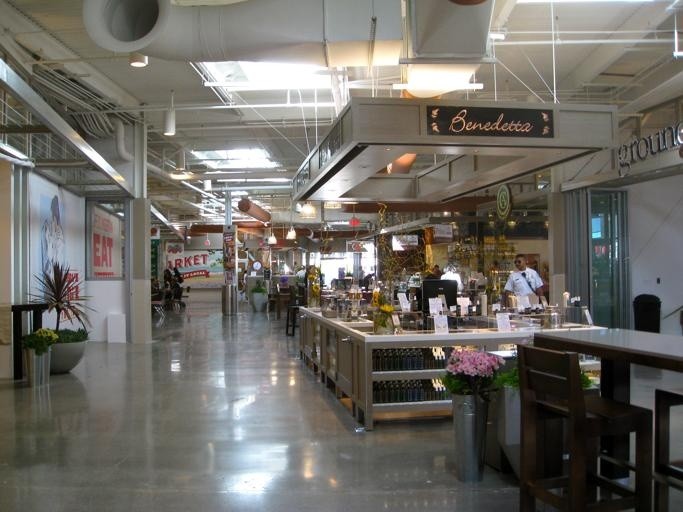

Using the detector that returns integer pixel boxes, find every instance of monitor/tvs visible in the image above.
[422,280,457,315]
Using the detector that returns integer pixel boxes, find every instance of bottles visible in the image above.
[467,294,488,316]
[411,294,418,311]
[372,348,444,369]
[322,283,396,335]
[524,306,539,314]
[398,275,420,291]
[544,306,561,327]
[372,380,450,403]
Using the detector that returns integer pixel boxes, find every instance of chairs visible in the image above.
[515,343,654,510]
[285,284,303,336]
[151,277,191,328]
[275,282,290,320]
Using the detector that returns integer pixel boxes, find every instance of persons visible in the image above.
[239,269,248,303]
[421,252,544,312]
[42,194,65,283]
[163,259,188,310]
[356,264,366,288]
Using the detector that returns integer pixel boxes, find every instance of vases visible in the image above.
[24,347,50,388]
[450,389,491,483]
[371,280,395,334]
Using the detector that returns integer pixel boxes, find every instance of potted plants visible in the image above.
[250,278,268,313]
[26,261,101,375]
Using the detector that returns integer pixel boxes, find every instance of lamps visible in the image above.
[128,52,148,68]
[160,91,178,137]
[267,193,341,246]
[175,148,185,171]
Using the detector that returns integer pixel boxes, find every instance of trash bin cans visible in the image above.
[222,283,238,316]
[633,294,661,332]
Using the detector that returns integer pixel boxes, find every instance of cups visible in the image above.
[448,306,457,316]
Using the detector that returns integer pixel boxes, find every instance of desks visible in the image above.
[532,325,681,511]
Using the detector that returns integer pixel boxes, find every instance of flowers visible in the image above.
[371,198,430,335]
[306,264,320,310]
[21,327,59,356]
[440,344,506,400]
